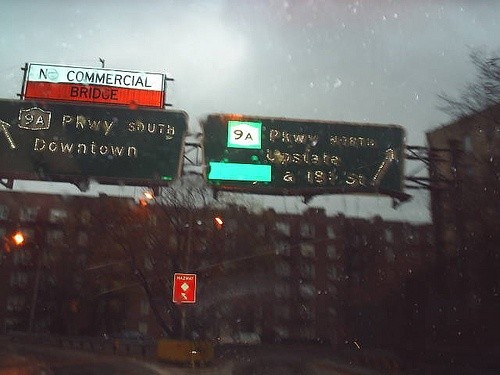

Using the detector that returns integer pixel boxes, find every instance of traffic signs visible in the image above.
[172,272,197,304]
[205,113,404,197]
[0,98,187,185]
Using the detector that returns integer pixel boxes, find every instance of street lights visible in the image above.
[13,232,43,338]
[214,216,229,338]
